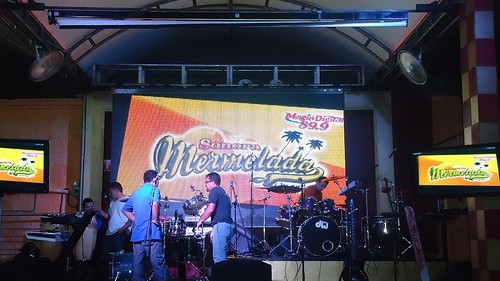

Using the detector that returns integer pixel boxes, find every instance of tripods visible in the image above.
[252,190,297,256]
[189,223,211,281]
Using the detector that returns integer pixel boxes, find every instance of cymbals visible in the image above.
[321,176,347,182]
[268,185,304,194]
[160,197,182,203]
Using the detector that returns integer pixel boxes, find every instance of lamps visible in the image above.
[398,39,432,85]
[43,7,410,28]
[29,39,65,83]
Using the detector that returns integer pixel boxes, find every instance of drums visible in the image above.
[297,215,342,257]
[162,220,187,237]
[275,205,297,227]
[108,252,134,281]
[175,235,212,264]
[182,194,208,216]
[318,197,337,216]
[297,195,318,215]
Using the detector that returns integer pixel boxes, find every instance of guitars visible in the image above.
[339,199,369,281]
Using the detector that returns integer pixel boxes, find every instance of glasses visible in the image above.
[205,181,211,183]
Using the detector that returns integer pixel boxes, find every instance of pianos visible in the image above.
[25,210,96,263]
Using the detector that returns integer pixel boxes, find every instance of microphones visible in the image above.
[383,178,393,183]
[298,177,303,187]
[230,179,234,186]
[153,174,161,182]
[389,147,398,158]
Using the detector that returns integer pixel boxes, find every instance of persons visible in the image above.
[82,197,103,229]
[93,182,132,257]
[300,177,329,202]
[121,171,168,281]
[196,172,233,263]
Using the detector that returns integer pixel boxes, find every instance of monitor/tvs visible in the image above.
[410,143,500,198]
[0,139,50,194]
